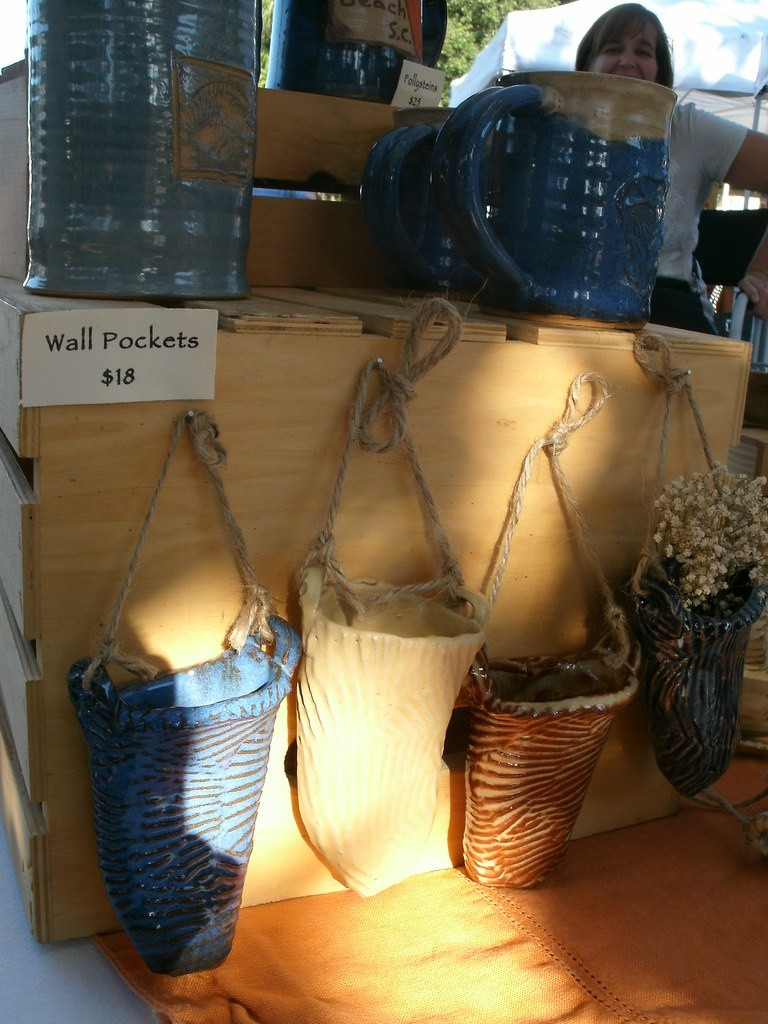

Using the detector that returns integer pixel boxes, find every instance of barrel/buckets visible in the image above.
[21,0,262,300]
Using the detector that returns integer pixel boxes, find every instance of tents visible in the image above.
[449,1,767,371]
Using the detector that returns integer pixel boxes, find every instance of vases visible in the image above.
[618,576,768,797]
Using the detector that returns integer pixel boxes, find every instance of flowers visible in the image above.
[636,462,768,618]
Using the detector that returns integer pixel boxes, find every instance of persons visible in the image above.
[575,4,768,335]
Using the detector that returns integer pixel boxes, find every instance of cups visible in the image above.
[363,108,494,291]
[432,70,676,330]
[265,0,447,104]
